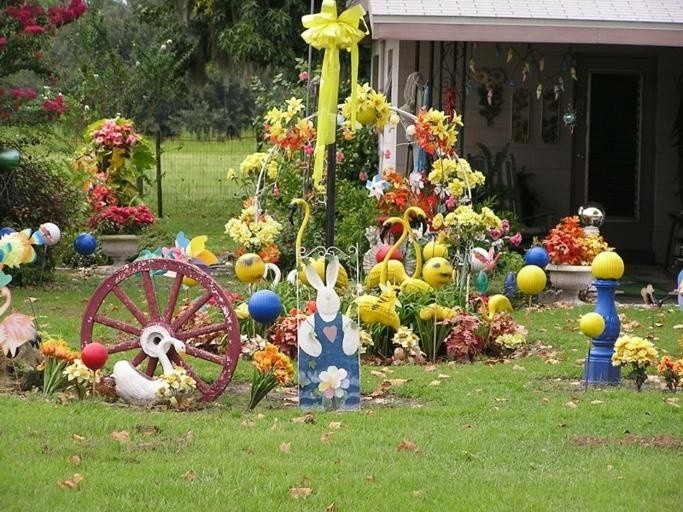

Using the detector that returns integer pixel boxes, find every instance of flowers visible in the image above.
[534,215,615,265]
[79,119,154,235]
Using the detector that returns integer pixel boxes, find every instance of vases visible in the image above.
[546,262,596,306]
[98,233,141,267]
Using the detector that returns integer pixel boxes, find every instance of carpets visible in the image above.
[609,264,678,296]
